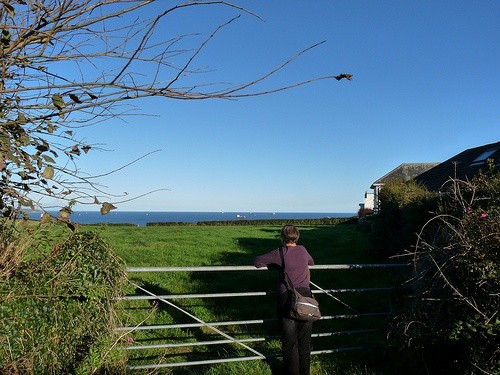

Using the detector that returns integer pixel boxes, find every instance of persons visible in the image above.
[254,225,314,375]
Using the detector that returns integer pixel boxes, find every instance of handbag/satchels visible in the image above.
[291,290,321,322]
